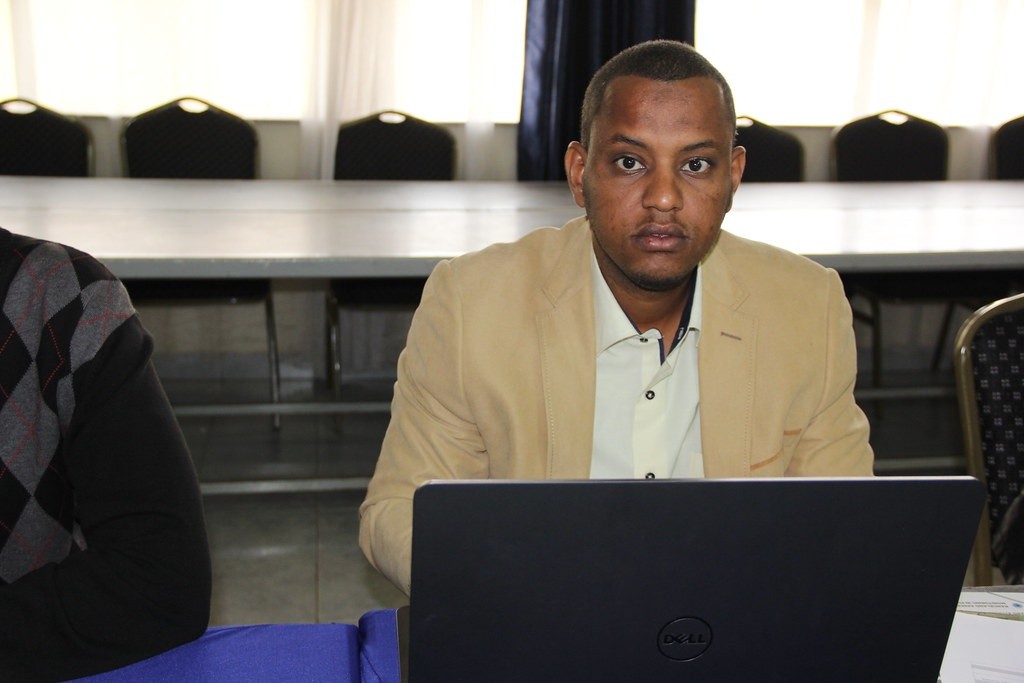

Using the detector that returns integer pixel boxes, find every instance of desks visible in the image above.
[56,584,1024,683]
[0,179,1024,495]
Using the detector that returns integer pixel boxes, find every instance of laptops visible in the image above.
[408,475,983,682]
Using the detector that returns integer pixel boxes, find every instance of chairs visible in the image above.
[324,111,460,436]
[119,98,281,427]
[955,292,1024,586]
[733,117,804,185]
[989,116,1024,180]
[827,108,973,390]
[0,99,93,180]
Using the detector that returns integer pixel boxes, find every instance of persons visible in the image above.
[359,39,876,593]
[0,230,213,683]
